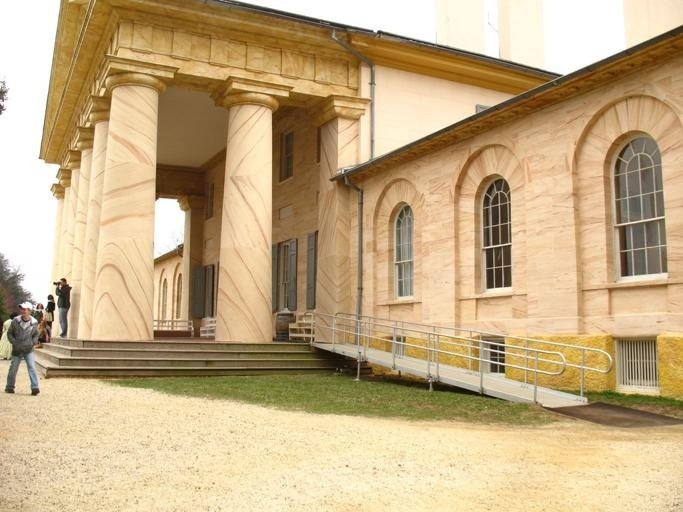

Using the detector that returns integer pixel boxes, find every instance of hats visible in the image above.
[19,302,33,310]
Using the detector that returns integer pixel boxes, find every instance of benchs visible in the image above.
[153,320,194,337]
[288,312,315,340]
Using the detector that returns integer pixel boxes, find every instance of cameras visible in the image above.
[54,282,61,285]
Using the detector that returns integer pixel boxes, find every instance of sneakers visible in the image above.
[32,389,40,395]
[5,388,14,393]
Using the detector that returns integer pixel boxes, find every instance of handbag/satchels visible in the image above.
[43,312,52,321]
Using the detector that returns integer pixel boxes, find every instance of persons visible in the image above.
[0,278,72,360]
[5,302,40,396]
